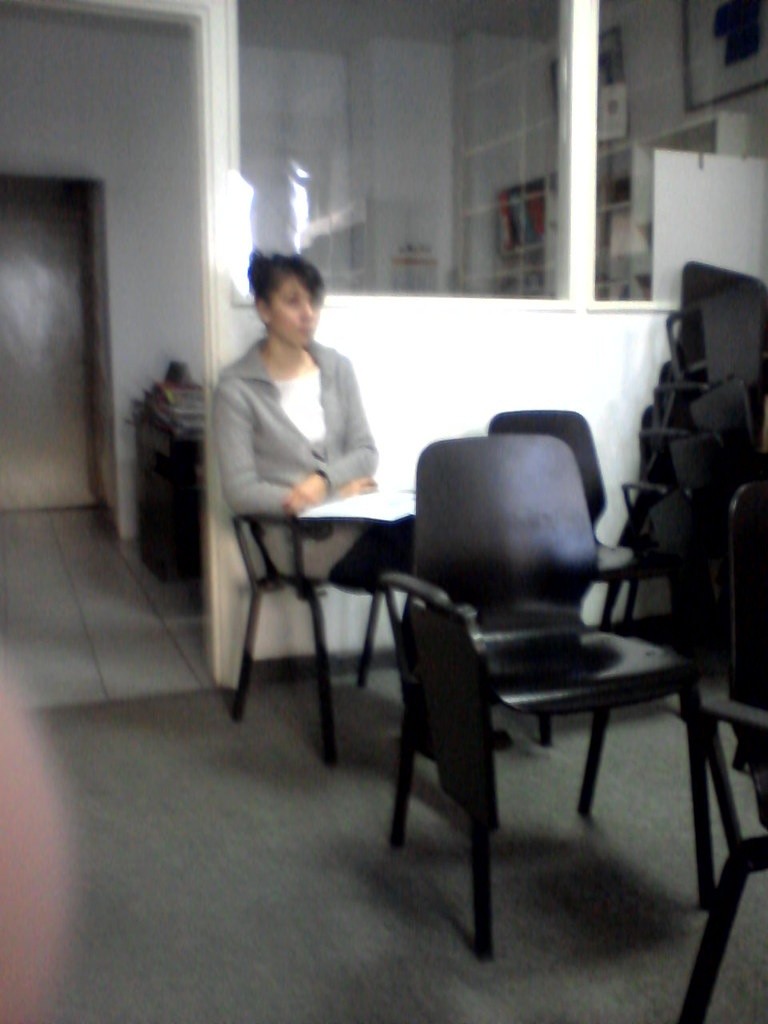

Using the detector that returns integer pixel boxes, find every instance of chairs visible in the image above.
[224,510,399,772]
[491,409,643,747]
[379,430,722,959]
[600,257,768,646]
[674,477,768,1024]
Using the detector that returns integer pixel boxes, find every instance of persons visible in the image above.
[215,247,514,756]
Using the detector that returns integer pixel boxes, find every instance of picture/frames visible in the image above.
[677,0,768,111]
